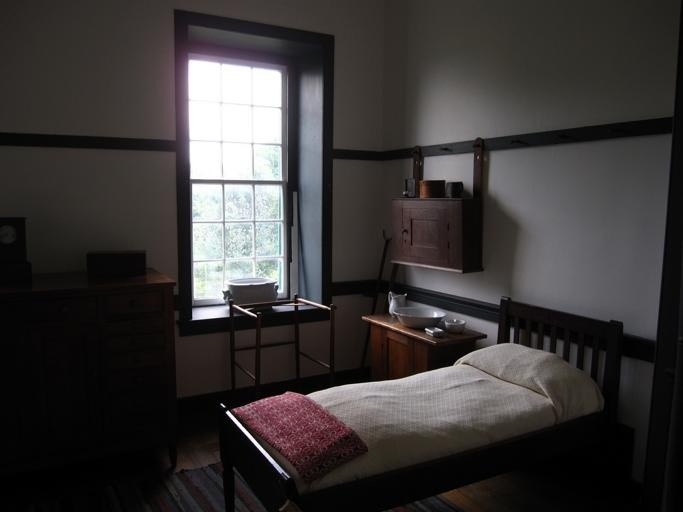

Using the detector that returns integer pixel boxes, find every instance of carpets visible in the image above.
[0,459,467,512]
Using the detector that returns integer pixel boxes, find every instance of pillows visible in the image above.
[453,341,604,423]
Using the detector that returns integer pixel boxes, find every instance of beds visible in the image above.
[217,296,623,511]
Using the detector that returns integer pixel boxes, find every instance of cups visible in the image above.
[445,181,463,198]
[388,291,407,317]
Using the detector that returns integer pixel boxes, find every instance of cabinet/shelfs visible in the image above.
[0,267,177,474]
[390,197,485,274]
[228,295,337,395]
[361,313,487,381]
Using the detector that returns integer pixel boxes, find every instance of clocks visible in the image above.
[0,216,33,287]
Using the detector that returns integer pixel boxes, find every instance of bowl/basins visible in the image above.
[222,277,280,310]
[444,319,466,333]
[392,307,447,328]
